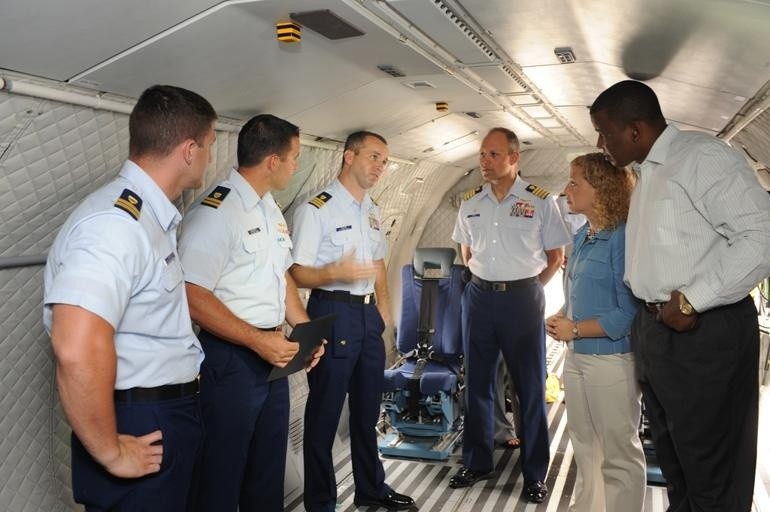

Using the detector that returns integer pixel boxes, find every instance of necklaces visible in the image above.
[587,226,602,240]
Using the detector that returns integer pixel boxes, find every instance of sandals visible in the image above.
[500,436,521,452]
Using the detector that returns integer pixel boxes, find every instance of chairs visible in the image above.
[378,248,467,461]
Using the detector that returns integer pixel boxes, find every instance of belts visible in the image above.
[468,273,541,293]
[113,375,205,404]
[643,302,666,313]
[309,288,379,307]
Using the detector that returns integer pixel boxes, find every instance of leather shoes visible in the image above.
[522,480,549,504]
[447,467,496,490]
[353,488,414,511]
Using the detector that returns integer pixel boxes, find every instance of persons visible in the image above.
[288,131,415,512]
[545,151,647,511]
[176,114,328,512]
[42,84,218,512]
[492,350,522,449]
[448,125,573,504]
[589,80,770,512]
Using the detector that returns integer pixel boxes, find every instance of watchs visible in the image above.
[677,292,698,317]
[572,320,582,340]
